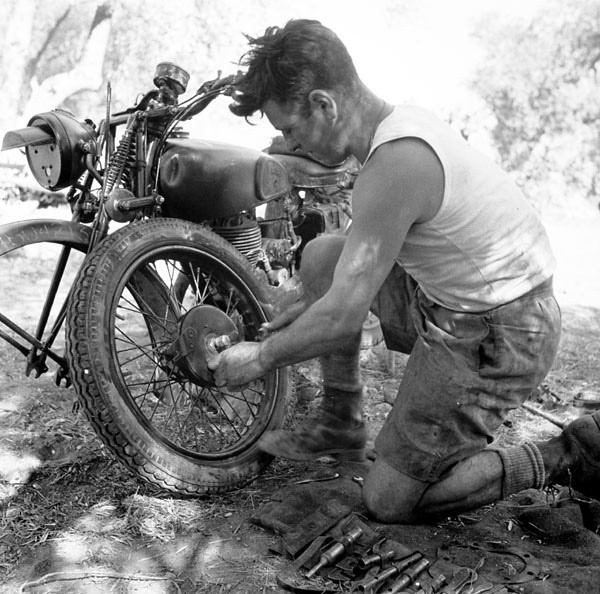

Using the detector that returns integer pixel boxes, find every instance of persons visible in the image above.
[207,20,599,535]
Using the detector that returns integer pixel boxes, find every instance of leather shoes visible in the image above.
[258,416,366,464]
[565,410,600,530]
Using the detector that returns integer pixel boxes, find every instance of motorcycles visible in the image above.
[0,60,384,390]
